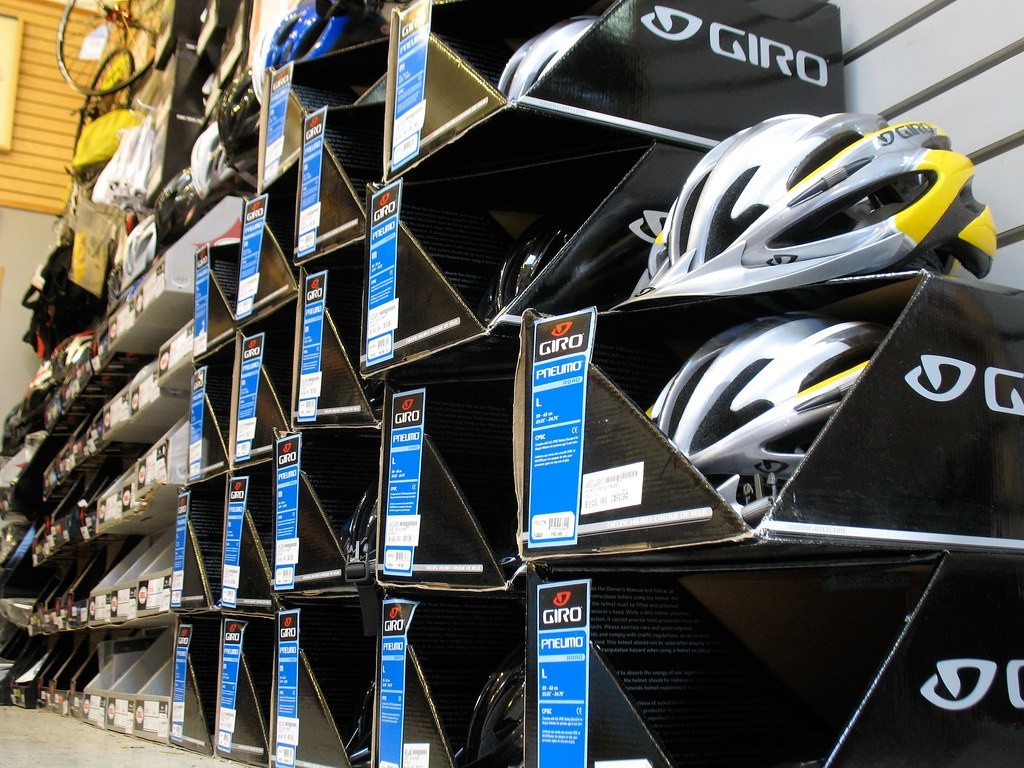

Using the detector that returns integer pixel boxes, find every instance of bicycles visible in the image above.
[55,0,178,154]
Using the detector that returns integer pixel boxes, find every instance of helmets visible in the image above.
[643,307,891,521]
[189,120,228,200]
[0,332,98,456]
[149,165,200,242]
[118,214,158,292]
[216,63,257,154]
[609,111,997,311]
[265,0,387,73]
[495,14,603,100]
[345,678,375,762]
[338,494,379,561]
[481,227,569,327]
[454,661,524,768]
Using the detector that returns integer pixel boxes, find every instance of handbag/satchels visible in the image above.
[91,114,156,212]
[22,244,113,359]
